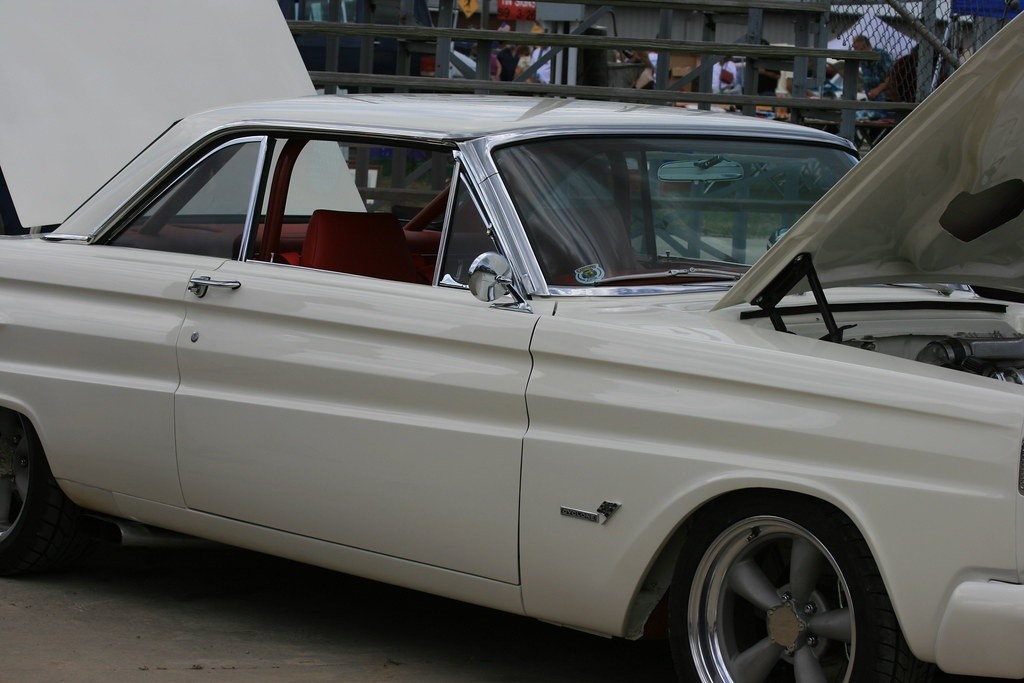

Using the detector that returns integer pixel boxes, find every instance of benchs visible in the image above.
[158,222,445,271]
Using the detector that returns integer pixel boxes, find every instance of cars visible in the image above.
[0,0,1024,683]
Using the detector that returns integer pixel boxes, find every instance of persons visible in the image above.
[647,50,658,89]
[851,37,892,149]
[731,39,782,112]
[883,45,918,125]
[459,23,552,86]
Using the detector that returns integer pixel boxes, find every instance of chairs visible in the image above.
[527,202,650,290]
[298,208,418,284]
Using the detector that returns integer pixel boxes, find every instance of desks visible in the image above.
[804,115,898,150]
